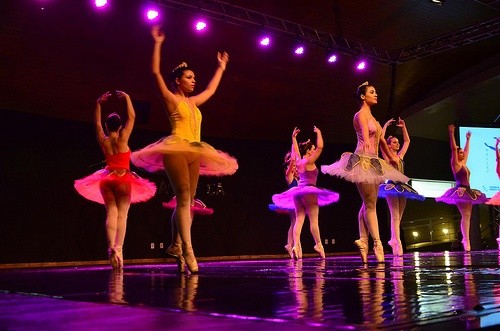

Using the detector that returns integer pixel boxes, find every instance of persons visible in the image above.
[376,117,410,256]
[268,143,303,259]
[435,124,487,252]
[320,80,410,262]
[130,25,238,274]
[273,126,340,259]
[486,136,500,250]
[73,90,157,269]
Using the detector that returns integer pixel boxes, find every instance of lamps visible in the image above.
[324,50,337,63]
[351,57,366,70]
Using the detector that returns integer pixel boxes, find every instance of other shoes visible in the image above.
[395,240,403,256]
[183,242,197,272]
[167,244,185,273]
[388,240,397,256]
[314,244,325,258]
[114,247,123,267]
[291,246,298,259]
[462,238,470,251]
[109,248,118,268]
[496,237,500,250]
[284,244,293,258]
[298,245,302,259]
[355,240,367,263]
[373,242,384,262]
